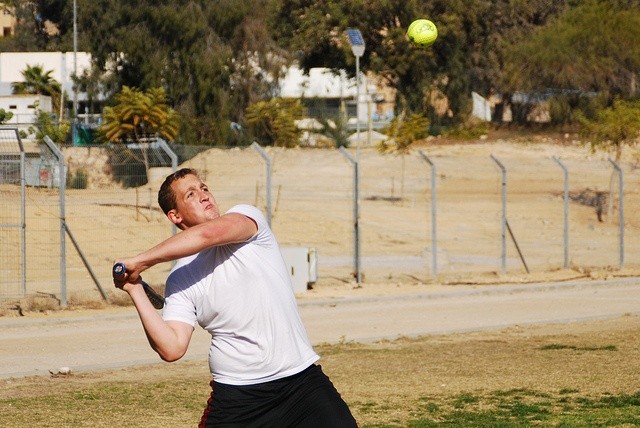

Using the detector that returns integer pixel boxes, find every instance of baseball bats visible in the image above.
[112,263,165,310]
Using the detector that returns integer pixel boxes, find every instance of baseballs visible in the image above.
[406,19,438,49]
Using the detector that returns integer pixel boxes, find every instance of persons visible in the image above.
[112,167,359,427]
